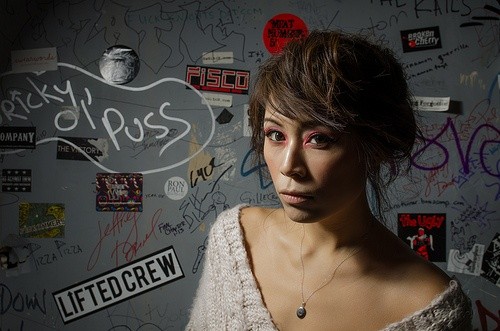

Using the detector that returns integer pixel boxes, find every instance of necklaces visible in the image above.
[294,221,389,318]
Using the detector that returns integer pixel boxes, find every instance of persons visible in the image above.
[174,30,479,331]
